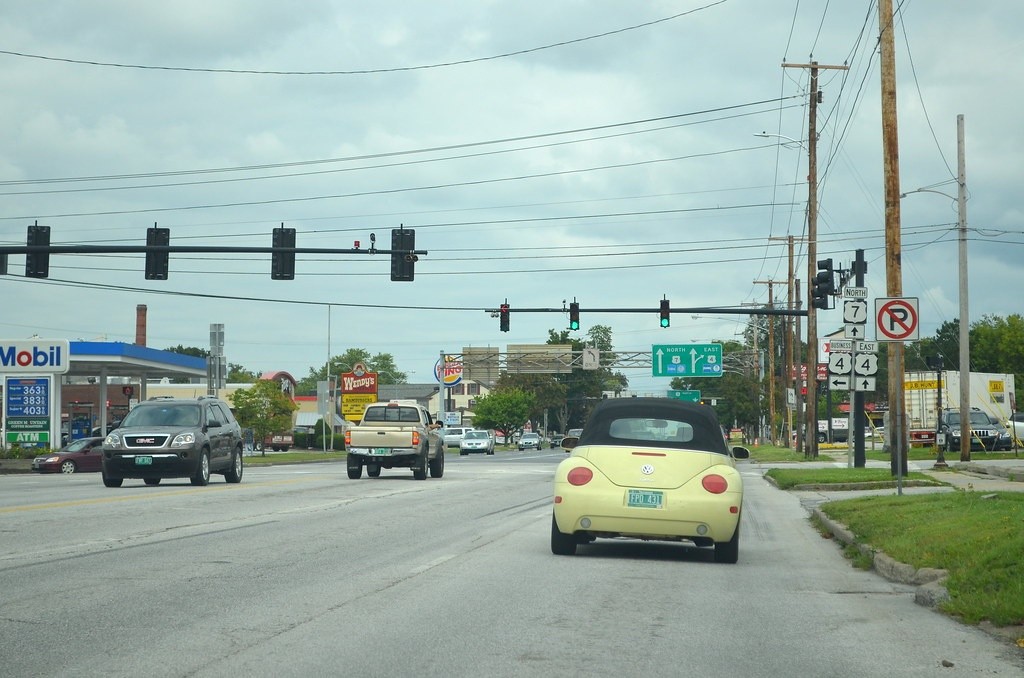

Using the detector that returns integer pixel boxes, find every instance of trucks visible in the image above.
[904,369,1016,448]
[802,417,855,443]
[247,432,294,452]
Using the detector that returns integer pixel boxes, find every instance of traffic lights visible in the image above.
[500,304,509,332]
[660,299,670,327]
[810,276,828,309]
[569,303,579,330]
[817,258,834,295]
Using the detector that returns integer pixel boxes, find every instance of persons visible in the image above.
[409,411,441,429]
[62,437,67,448]
[89,443,97,450]
[173,407,198,426]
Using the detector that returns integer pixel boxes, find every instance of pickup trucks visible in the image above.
[345,402,444,480]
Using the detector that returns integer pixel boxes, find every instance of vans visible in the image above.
[445,428,476,447]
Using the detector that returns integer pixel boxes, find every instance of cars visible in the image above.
[518,433,542,451]
[92,426,112,437]
[102,396,243,487]
[460,431,494,455]
[865,427,884,438]
[989,412,1024,451]
[551,398,749,563]
[549,429,583,453]
[31,437,106,474]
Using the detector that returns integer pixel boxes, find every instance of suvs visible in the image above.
[941,407,1000,452]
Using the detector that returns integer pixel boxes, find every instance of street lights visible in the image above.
[900,190,971,461]
[377,371,398,399]
[755,132,816,459]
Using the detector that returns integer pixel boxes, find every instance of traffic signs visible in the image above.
[652,344,723,377]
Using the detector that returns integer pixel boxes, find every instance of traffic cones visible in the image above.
[754,438,758,447]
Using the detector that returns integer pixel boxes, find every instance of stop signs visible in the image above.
[667,390,701,403]
